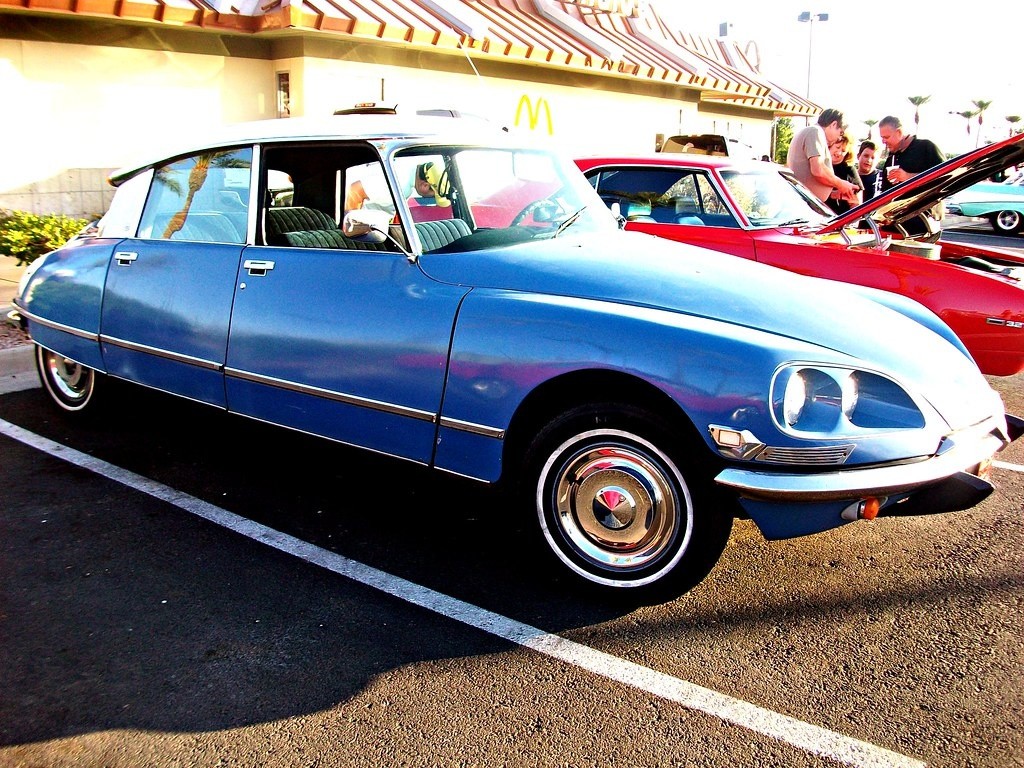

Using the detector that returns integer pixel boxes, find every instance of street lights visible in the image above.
[797,10,829,99]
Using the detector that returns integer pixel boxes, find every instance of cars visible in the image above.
[942,164,1024,238]
[5,116,1024,608]
[394,129,1024,377]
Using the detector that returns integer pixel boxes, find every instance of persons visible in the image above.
[344,159,451,222]
[787,108,859,203]
[824,135,882,230]
[879,116,947,239]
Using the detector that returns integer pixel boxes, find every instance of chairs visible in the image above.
[667,196,707,226]
[622,195,656,224]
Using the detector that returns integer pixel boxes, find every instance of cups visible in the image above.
[886,165,900,183]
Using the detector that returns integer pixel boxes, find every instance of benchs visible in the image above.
[167,207,343,244]
[283,216,476,253]
